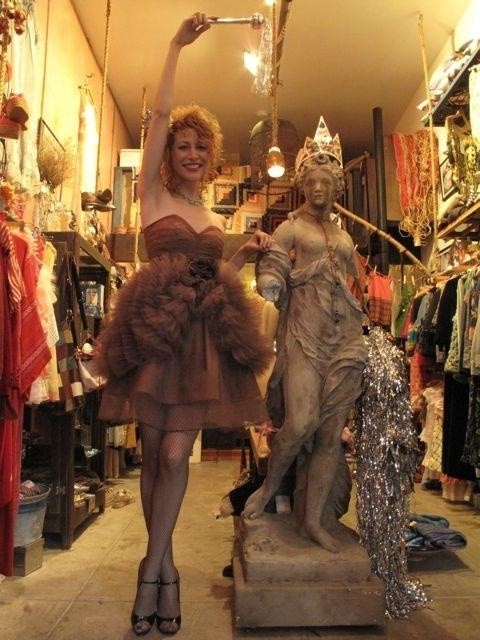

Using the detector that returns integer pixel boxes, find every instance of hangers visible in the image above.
[418,249,479,287]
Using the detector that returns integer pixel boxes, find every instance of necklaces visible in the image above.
[164,183,205,206]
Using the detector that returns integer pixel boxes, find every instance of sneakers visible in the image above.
[6,92,29,132]
[103,487,116,508]
[112,488,136,510]
[1,111,21,139]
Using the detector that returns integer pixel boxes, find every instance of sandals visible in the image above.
[156,566,183,637]
[131,557,160,636]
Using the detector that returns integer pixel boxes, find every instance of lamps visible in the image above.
[265,16,287,180]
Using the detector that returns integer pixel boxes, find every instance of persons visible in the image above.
[87,9,278,637]
[237,113,376,551]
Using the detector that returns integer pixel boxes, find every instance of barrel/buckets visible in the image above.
[12,482,52,549]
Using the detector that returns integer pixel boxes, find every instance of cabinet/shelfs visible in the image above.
[21,230,113,552]
[422,43,480,240]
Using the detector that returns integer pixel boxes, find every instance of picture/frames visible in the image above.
[36,114,68,201]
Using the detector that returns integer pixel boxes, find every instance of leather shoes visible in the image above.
[97,188,113,204]
[80,191,116,211]
